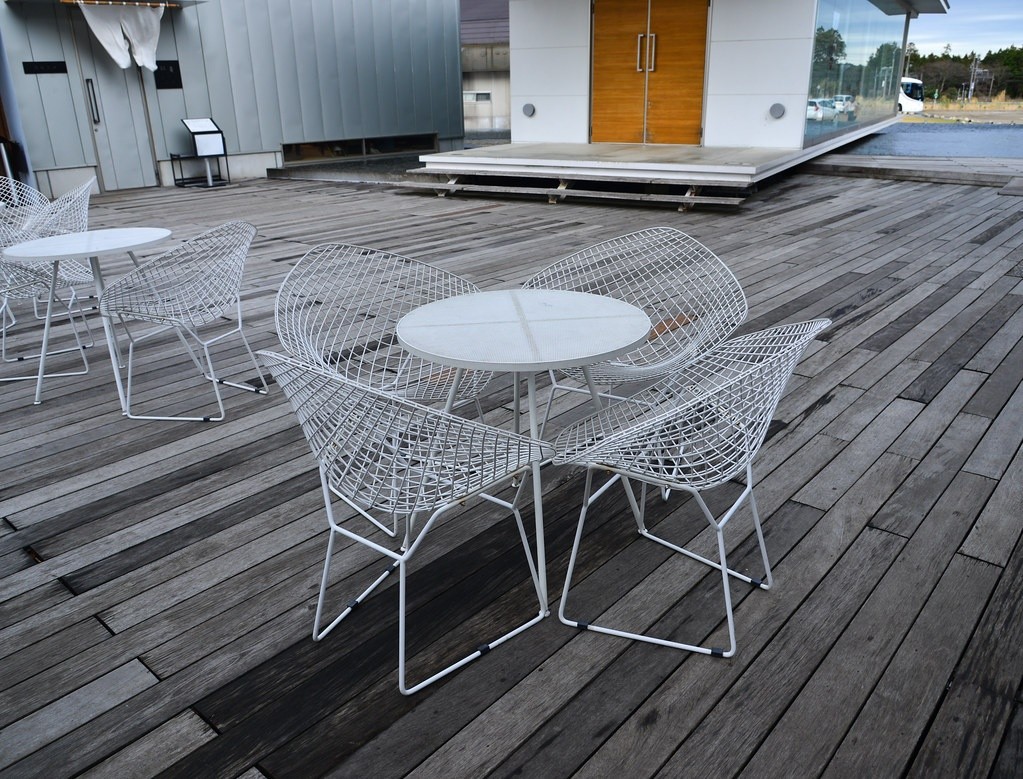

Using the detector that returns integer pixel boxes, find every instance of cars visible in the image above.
[807,98,839,122]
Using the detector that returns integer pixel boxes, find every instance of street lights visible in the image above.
[888,44,899,97]
[906,54,911,77]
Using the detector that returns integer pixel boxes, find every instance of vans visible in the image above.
[832,94,855,113]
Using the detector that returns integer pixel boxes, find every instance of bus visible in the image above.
[897,76,925,113]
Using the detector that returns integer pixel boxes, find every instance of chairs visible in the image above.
[273,242,492,538]
[0,176,95,381]
[19,175,100,319]
[254,350,545,694]
[100,220,269,420]
[510,226,748,501]
[552,317,832,657]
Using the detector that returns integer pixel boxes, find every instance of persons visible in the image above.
[843,95,860,121]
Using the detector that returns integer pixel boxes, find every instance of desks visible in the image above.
[397,289,651,615]
[3,227,172,405]
